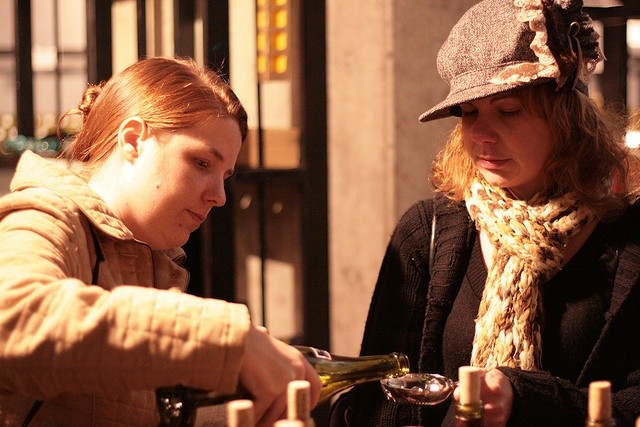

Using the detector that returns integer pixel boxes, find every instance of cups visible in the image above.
[154,345,409,427]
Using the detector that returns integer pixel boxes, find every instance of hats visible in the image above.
[418,0,609,122]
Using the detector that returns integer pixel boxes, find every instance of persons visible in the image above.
[0,54,323,427]
[347,1,640,427]
